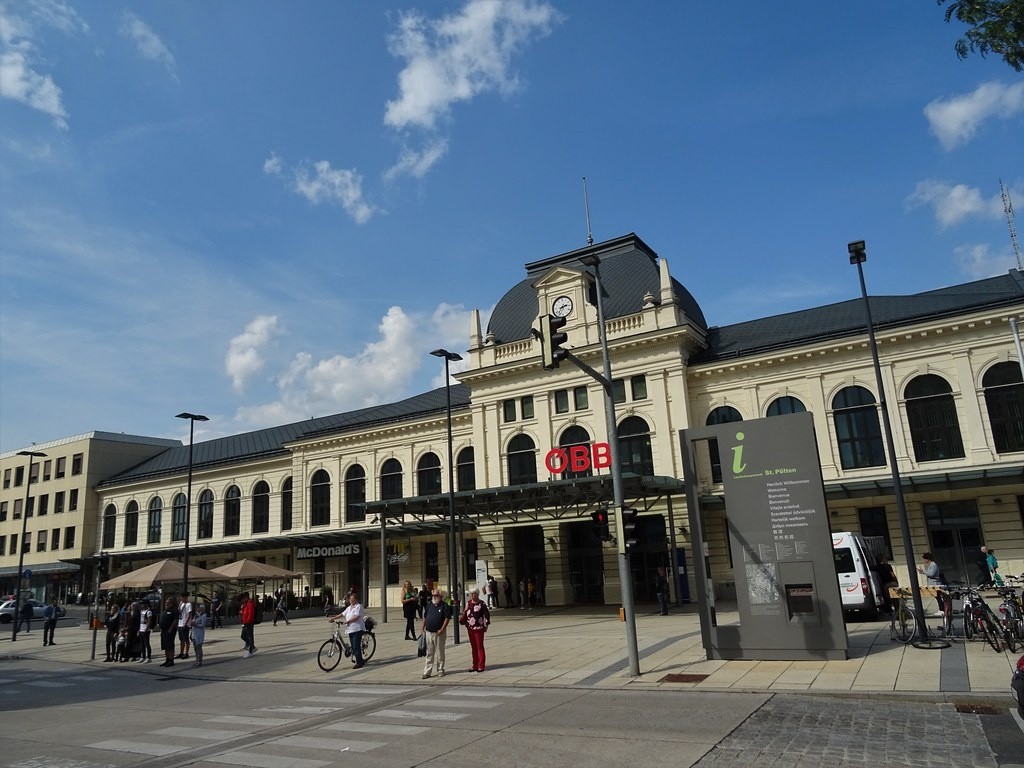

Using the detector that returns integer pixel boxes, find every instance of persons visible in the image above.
[485,575,537,611]
[210,591,223,630]
[16,598,34,633]
[976,545,998,589]
[42,599,58,646]
[103,600,153,664]
[238,592,260,658]
[916,552,940,586]
[158,593,207,667]
[63,590,127,610]
[655,572,669,615]
[875,552,899,620]
[401,580,490,679]
[261,583,311,626]
[328,584,367,669]
[6,592,14,600]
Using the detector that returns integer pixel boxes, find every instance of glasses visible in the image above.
[431,595,438,597]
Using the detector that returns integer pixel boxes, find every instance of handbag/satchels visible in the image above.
[993,573,1003,587]
[417,637,427,657]
[459,612,467,625]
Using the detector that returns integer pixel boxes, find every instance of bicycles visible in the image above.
[888,586,917,645]
[317,619,376,671]
[936,574,1024,653]
[910,624,951,650]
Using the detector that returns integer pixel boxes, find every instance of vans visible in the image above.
[831,531,886,621]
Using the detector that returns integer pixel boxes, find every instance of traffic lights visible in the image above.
[539,313,570,372]
[613,504,639,555]
[590,510,611,541]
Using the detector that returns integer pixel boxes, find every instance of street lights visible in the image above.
[577,253,642,678]
[11,450,48,642]
[847,238,930,641]
[429,348,463,644]
[175,411,211,593]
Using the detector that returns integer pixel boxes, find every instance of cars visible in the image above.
[0,599,67,625]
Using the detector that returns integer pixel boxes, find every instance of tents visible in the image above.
[99,559,302,616]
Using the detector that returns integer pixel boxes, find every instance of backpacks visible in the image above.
[145,609,157,629]
[248,600,263,624]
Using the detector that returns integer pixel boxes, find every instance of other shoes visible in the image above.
[243,651,253,658]
[405,636,412,640]
[468,669,474,672]
[477,669,483,672]
[251,648,257,652]
[104,652,202,666]
[43,642,46,646]
[352,664,362,669]
[49,642,56,645]
[286,622,290,625]
[422,675,430,679]
[413,638,416,641]
[438,672,444,676]
[273,622,277,626]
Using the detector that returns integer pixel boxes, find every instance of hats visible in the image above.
[922,553,934,562]
